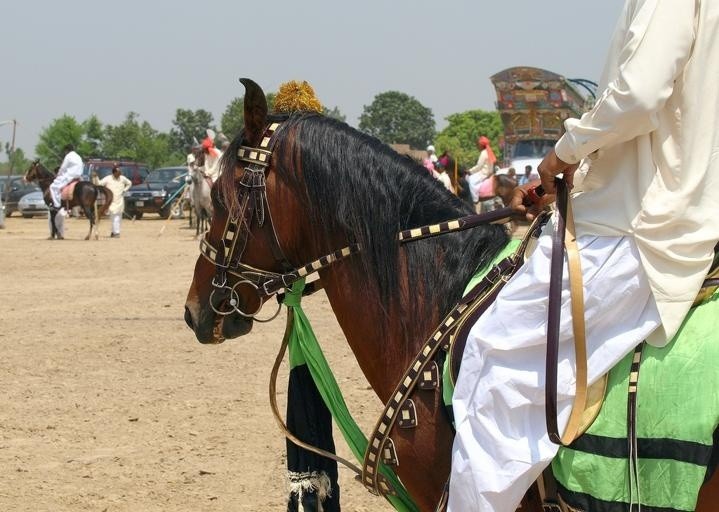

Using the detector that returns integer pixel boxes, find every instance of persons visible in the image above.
[46,167,66,240]
[185,143,203,187]
[201,138,225,185]
[92,162,133,240]
[48,142,84,210]
[424,132,533,216]
[444,2,718,511]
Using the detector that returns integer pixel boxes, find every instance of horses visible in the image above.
[25,158,113,240]
[434,150,519,233]
[183,77,719,512]
[184,158,213,236]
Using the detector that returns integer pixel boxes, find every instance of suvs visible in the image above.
[0,158,197,220]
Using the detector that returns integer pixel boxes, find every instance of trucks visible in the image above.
[493,134,573,196]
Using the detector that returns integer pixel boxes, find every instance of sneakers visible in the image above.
[111,232,119,238]
